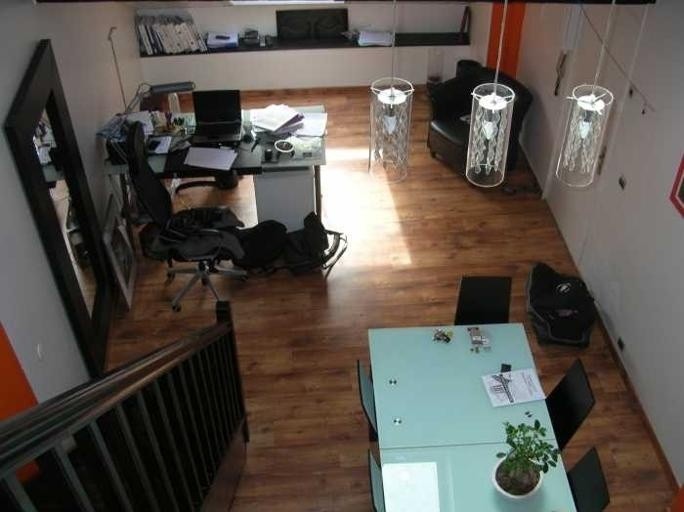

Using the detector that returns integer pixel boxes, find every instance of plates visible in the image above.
[274,140,294,154]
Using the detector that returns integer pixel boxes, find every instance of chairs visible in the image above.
[357,359,376,440]
[525,256,598,350]
[455,274,514,325]
[364,450,387,512]
[543,358,595,456]
[566,445,612,511]
[125,121,247,316]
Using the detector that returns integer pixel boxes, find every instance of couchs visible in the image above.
[424,59,534,183]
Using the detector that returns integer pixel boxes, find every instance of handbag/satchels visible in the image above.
[526,263,594,347]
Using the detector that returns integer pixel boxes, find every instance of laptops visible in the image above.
[192,89,241,144]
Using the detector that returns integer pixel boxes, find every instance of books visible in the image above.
[134,12,240,55]
[358,29,394,46]
[480,367,548,408]
[248,103,300,136]
[252,113,305,134]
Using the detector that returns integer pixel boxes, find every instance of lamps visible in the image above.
[553,3,617,190]
[367,2,417,186]
[466,1,514,189]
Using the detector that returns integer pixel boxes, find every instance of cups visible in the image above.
[243,122,252,136]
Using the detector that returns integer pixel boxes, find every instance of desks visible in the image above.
[379,444,583,512]
[366,322,560,449]
[107,103,339,253]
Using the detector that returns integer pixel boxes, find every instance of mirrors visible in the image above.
[2,38,120,374]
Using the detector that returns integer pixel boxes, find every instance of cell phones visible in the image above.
[471,328,481,343]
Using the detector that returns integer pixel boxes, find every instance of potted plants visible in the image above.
[488,411,564,503]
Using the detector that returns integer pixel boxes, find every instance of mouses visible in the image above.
[265,148,272,160]
